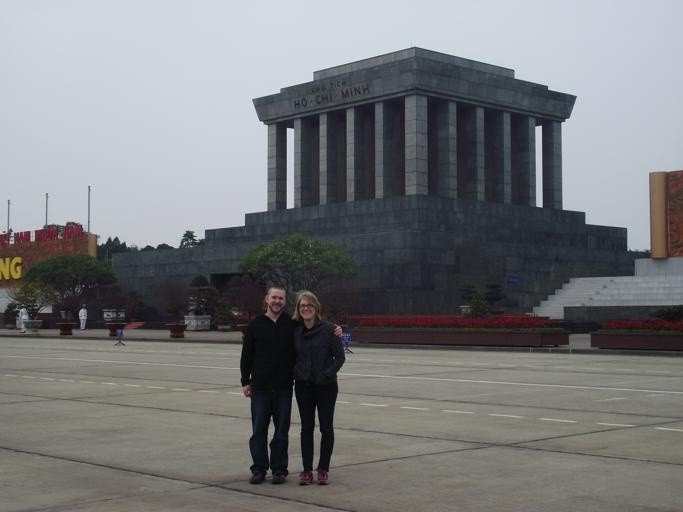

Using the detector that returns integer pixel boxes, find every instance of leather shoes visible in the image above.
[272,474,288,485]
[251,472,265,484]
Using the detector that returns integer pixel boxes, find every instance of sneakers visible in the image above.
[298,470,312,485]
[318,469,329,485]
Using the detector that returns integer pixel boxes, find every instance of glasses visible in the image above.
[299,304,314,307]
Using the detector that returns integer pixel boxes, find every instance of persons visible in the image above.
[239,285,342,484]
[290,289,346,485]
[18,303,29,333]
[78,303,88,330]
